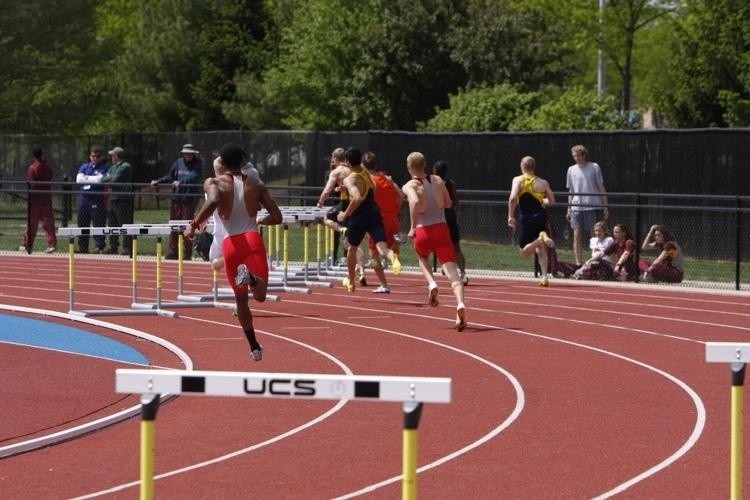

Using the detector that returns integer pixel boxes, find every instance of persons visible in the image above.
[402,151,466,332]
[316,148,405,293]
[433,160,466,287]
[571,221,640,282]
[181,141,283,363]
[507,156,555,287]
[16,147,57,256]
[205,151,265,317]
[639,225,683,283]
[74,148,134,254]
[567,145,608,276]
[150,144,202,260]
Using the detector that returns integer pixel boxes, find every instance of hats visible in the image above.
[181,143,200,154]
[108,147,124,154]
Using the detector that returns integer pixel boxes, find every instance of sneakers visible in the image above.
[373,287,391,293]
[359,274,367,286]
[92,248,100,254]
[74,247,88,253]
[235,264,249,288]
[250,344,263,360]
[99,247,119,254]
[391,254,402,274]
[343,277,355,292]
[462,277,469,286]
[46,247,56,254]
[539,279,549,286]
[428,285,440,307]
[455,305,467,332]
[539,231,555,248]
[120,248,132,255]
[20,246,32,254]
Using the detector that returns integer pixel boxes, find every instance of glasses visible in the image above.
[91,155,100,158]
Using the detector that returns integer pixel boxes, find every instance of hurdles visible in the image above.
[255,215,312,294]
[122,222,236,310]
[169,219,281,301]
[115,366,451,500]
[706,341,750,500]
[261,208,347,286]
[278,206,347,274]
[258,211,332,290]
[57,227,177,318]
